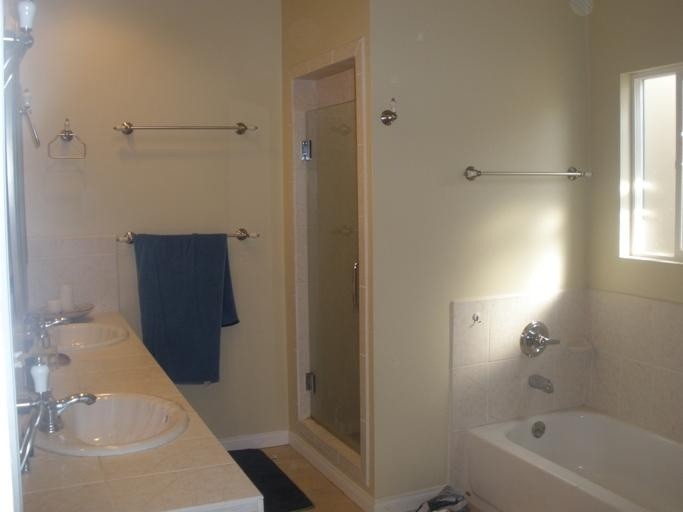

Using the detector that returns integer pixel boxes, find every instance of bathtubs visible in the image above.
[463,404,681,512]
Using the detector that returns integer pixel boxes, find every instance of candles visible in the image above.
[48,299,61,314]
[59,284,73,313]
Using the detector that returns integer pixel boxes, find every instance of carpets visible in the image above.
[228,448,314,512]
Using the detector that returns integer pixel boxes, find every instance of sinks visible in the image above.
[34,389,189,457]
[39,320,129,352]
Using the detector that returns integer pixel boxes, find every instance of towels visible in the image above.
[133,233,240,384]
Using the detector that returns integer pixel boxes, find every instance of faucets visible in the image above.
[34,313,75,350]
[38,389,97,435]
[528,372,556,394]
